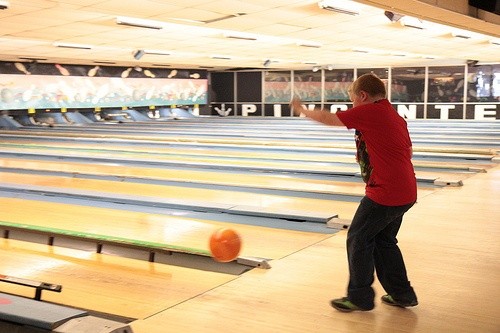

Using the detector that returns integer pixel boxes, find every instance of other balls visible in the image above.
[210,228,241,261]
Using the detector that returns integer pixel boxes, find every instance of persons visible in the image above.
[291,73,419,313]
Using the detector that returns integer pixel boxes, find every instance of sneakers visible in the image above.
[381,294,418,307]
[332,296,375,314]
